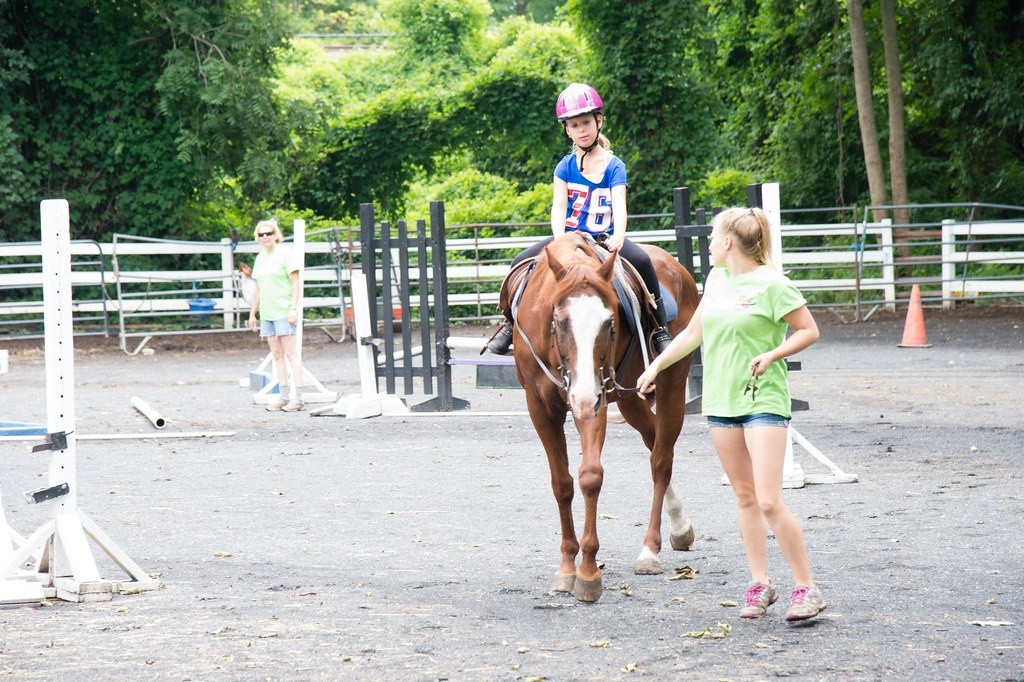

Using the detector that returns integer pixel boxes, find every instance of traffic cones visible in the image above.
[897,284,934,349]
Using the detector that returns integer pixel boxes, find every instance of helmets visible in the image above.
[555,82,604,123]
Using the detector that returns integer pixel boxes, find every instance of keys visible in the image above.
[743,365,760,402]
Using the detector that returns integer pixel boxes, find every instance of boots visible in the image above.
[645,296,673,354]
[486,324,515,355]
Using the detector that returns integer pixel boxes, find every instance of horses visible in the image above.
[499,234,700,603]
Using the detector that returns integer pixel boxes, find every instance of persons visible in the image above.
[486,83,675,358]
[634,204,830,621]
[248,219,308,413]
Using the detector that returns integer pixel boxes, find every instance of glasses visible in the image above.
[256,230,273,237]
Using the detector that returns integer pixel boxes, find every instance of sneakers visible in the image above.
[265,398,290,411]
[738,578,778,620]
[280,402,306,412]
[784,586,828,621]
